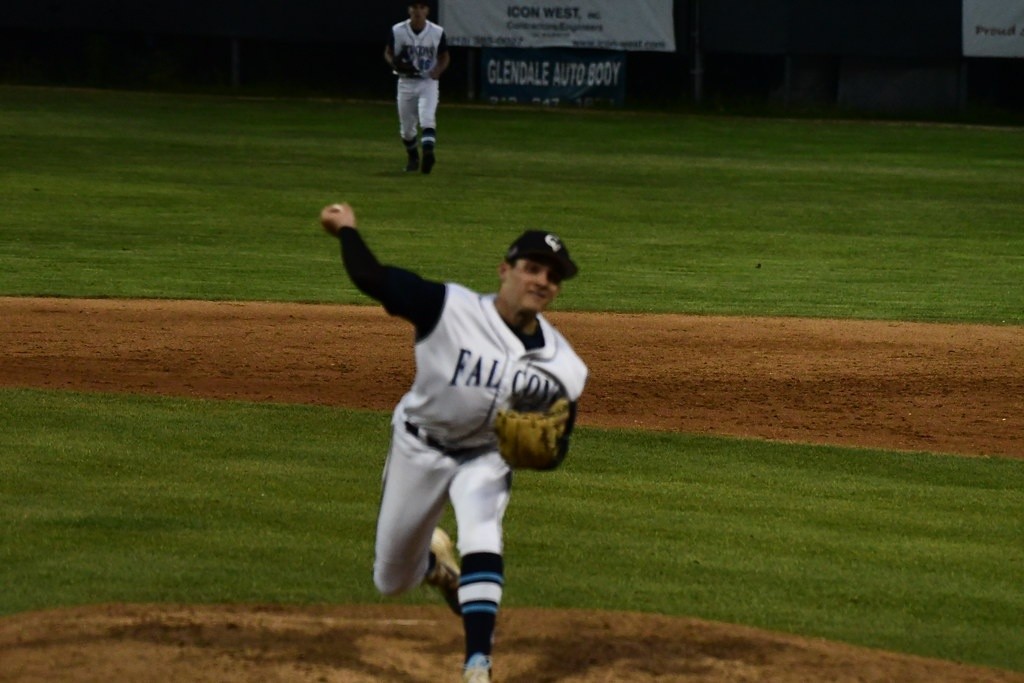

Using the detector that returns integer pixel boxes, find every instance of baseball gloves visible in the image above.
[494,392,577,471]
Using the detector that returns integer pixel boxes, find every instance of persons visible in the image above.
[385,0,449,174]
[320,201,589,683]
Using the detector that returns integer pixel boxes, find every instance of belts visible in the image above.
[405,420,497,460]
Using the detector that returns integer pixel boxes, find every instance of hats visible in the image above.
[504,229,576,279]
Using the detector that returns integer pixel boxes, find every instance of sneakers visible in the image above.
[421,153,434,175]
[427,527,461,614]
[459,655,494,683]
[407,153,419,171]
